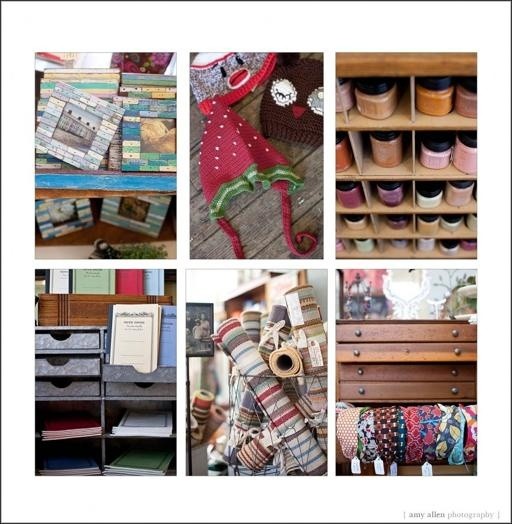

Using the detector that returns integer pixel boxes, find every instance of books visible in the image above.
[38,458,101,476]
[44,269,165,295]
[43,422,103,441]
[103,451,175,476]
[111,409,174,437]
[104,303,178,375]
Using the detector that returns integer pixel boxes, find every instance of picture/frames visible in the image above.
[100,197,172,240]
[35,198,95,240]
[45,269,71,294]
[36,68,177,192]
[186,303,215,358]
[71,270,116,294]
[116,270,144,296]
[144,269,165,296]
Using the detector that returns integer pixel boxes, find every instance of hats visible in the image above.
[259,60,324,148]
[197,94,318,257]
[191,53,279,115]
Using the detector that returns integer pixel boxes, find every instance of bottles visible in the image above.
[456,75,477,119]
[355,79,397,119]
[453,130,476,174]
[336,78,353,112]
[417,238,435,251]
[417,215,440,235]
[336,181,362,208]
[387,214,408,229]
[370,132,403,167]
[440,241,460,256]
[419,134,453,169]
[461,239,477,250]
[377,181,404,207]
[445,180,474,207]
[392,239,408,249]
[416,183,443,208]
[343,214,368,230]
[336,240,344,251]
[467,214,477,231]
[336,131,353,172]
[354,239,374,253]
[416,76,454,117]
[440,214,464,231]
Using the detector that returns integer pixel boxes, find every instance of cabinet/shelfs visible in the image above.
[34,325,177,475]
[37,292,172,329]
[335,319,477,476]
[336,52,477,259]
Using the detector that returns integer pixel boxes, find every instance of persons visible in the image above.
[192,319,201,340]
[200,312,210,338]
